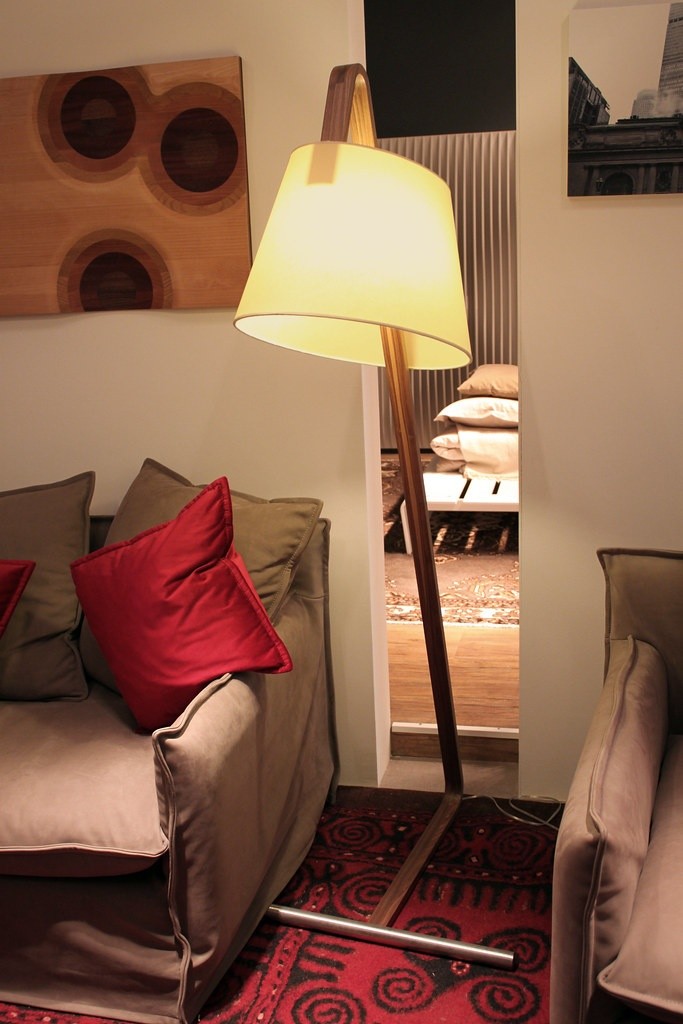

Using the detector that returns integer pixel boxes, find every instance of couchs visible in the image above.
[0,510,344,1024]
[544,547,683,1023]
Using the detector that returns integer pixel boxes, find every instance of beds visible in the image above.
[399,454,519,555]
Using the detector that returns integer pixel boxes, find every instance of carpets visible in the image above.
[380,461,519,626]
[0,784,569,1023]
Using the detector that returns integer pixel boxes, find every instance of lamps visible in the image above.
[229,64,519,970]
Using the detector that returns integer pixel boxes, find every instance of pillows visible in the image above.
[67,476,294,732]
[433,396,518,428]
[1,470,96,702]
[78,457,324,694]
[456,362,518,401]
